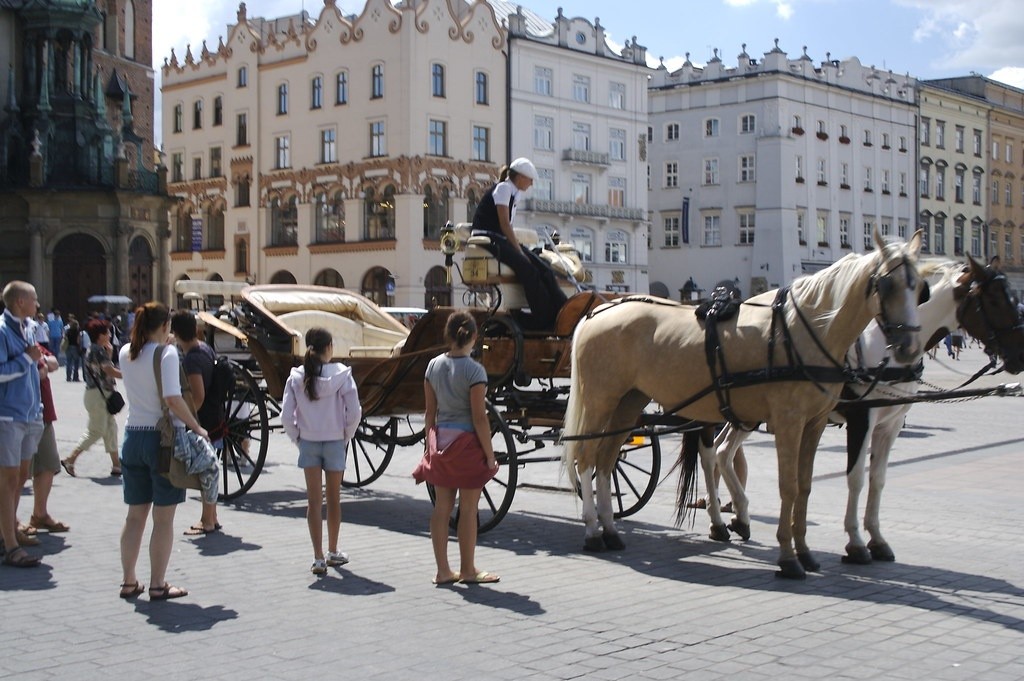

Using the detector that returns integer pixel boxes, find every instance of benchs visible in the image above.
[276,308,404,359]
[469,225,586,310]
[192,308,259,370]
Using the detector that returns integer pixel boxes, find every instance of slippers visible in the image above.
[433,570,500,584]
[183,524,223,535]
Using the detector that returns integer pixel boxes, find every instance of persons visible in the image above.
[119,300,210,600]
[165,314,250,468]
[471,158,569,340]
[930,328,983,360]
[687,426,748,512]
[280,327,362,572]
[61,320,123,476]
[413,311,500,584]
[0,280,70,567]
[171,310,223,535]
[33,308,136,382]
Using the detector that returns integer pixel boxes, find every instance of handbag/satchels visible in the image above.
[157,425,202,489]
[105,390,126,416]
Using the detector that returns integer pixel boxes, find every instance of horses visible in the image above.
[559,229,926,580]
[656,249,1024,566]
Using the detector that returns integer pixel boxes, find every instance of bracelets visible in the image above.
[518,252,525,255]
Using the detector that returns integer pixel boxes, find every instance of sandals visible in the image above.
[120,581,146,598]
[16,531,43,546]
[0,547,41,567]
[16,521,37,536]
[30,514,70,531]
[148,581,188,599]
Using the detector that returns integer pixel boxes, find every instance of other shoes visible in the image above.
[327,551,349,566]
[110,462,123,473]
[311,558,328,574]
[61,457,76,478]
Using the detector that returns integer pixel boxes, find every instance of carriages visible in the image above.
[173,219,1024,577]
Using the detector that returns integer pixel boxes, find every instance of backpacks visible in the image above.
[200,344,237,406]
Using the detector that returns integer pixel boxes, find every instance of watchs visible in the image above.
[42,354,48,363]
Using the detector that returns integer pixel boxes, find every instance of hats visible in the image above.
[510,158,540,180]
